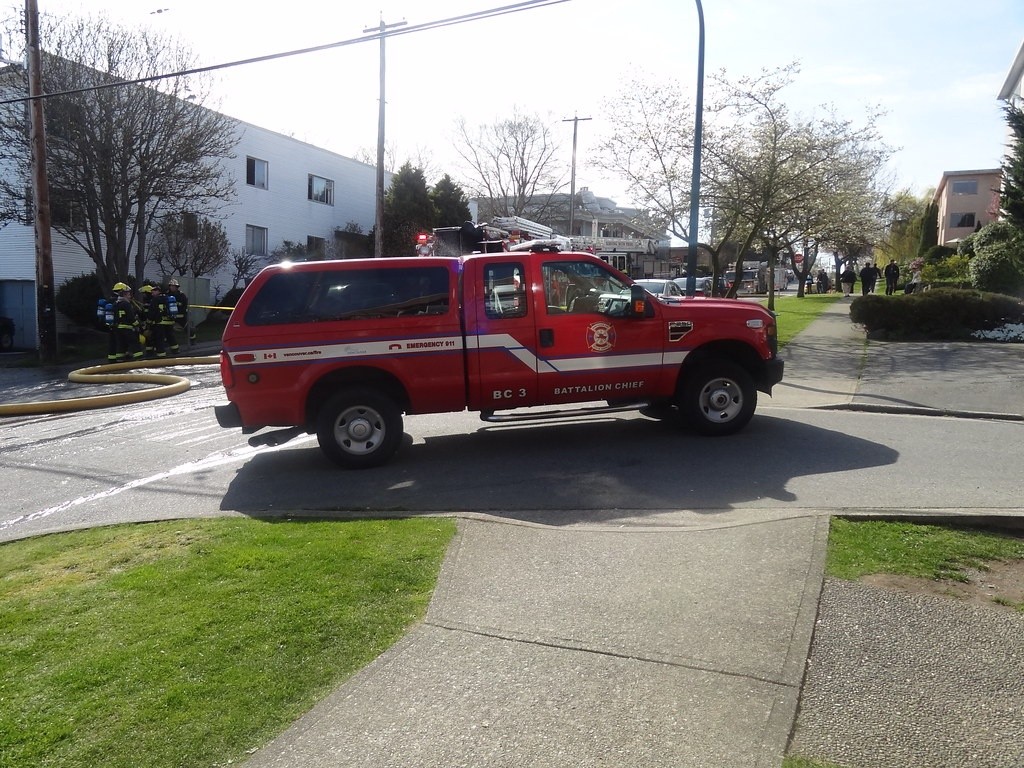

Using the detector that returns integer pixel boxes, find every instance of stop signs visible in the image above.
[795,254,804,263]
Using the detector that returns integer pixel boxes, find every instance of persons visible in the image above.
[106,279,195,362]
[807,270,828,294]
[884,259,899,294]
[894,261,899,293]
[840,265,856,297]
[871,263,882,293]
[905,267,922,294]
[860,262,873,295]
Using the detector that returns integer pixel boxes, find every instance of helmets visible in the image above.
[139,285,156,292]
[169,279,180,287]
[112,282,131,291]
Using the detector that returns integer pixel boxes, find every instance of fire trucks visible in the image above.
[417,217,659,315]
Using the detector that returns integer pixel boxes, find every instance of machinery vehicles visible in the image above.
[725,261,768,297]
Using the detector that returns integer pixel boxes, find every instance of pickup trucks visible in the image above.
[213,216,785,470]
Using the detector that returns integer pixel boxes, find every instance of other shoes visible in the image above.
[191,339,198,345]
[174,351,181,354]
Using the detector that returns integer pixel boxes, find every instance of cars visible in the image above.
[674,278,712,297]
[620,279,686,297]
[704,277,738,299]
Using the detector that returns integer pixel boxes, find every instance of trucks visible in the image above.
[768,268,788,291]
